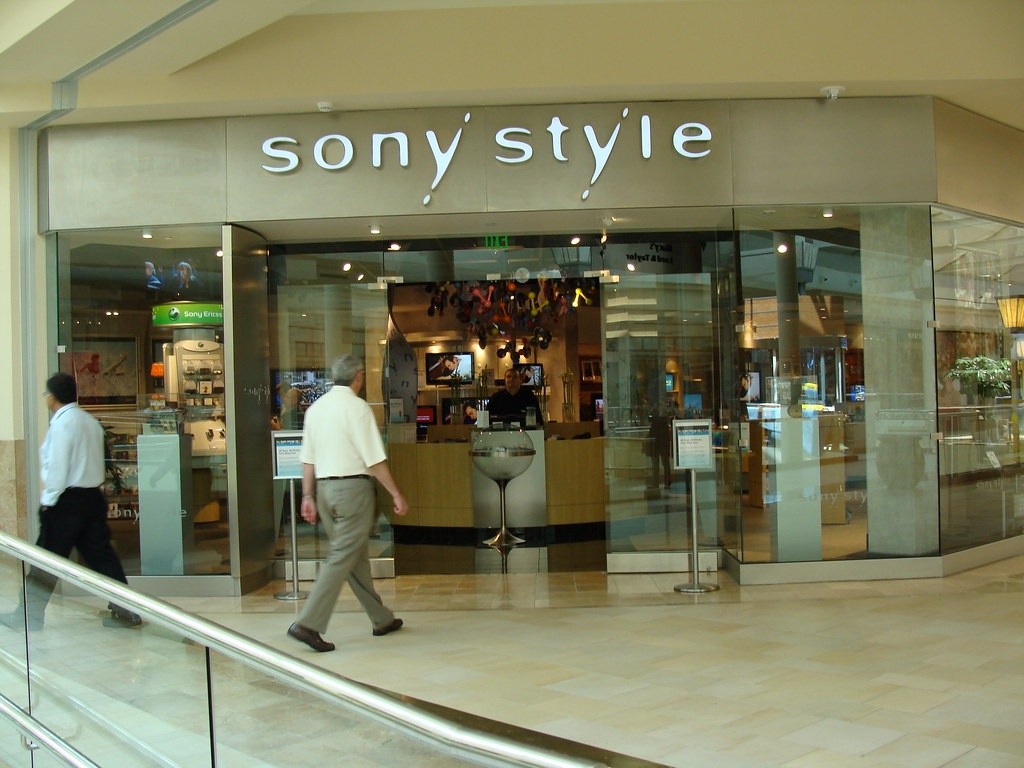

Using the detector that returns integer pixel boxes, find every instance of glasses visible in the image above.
[42,392,52,398]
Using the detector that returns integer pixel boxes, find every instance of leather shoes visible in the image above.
[288,622,335,652]
[373,618,403,636]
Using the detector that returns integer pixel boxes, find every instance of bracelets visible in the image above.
[302,494,314,498]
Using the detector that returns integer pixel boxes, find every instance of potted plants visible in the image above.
[533,368,548,423]
[559,367,576,424]
[449,367,462,425]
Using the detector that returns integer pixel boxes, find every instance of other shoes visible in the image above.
[111,608,142,626]
[1,612,44,632]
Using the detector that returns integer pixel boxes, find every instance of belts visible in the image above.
[318,474,369,479]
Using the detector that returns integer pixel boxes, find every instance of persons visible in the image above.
[429,355,471,379]
[956,250,998,364]
[270,381,301,430]
[647,397,681,491]
[78,354,105,376]
[521,365,541,385]
[145,262,196,300]
[286,353,410,652]
[464,402,478,424]
[739,372,760,402]
[0,372,141,631]
[485,368,544,431]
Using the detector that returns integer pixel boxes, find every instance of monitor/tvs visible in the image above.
[741,370,762,402]
[426,352,475,387]
[513,363,544,390]
[272,368,335,426]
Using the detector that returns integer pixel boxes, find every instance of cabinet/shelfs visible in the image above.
[748,412,849,508]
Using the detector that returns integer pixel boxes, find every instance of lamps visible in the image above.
[992,294,1024,333]
